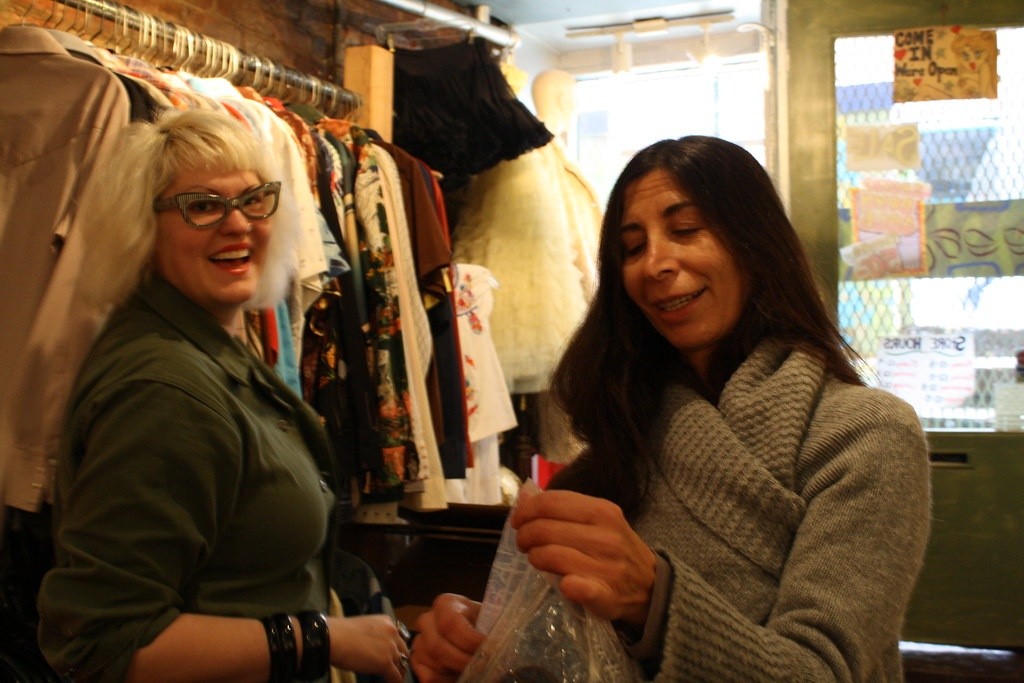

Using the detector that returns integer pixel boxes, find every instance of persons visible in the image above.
[35,110,413,683]
[529,69,605,469]
[407,135,932,683]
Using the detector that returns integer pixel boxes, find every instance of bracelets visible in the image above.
[293,610,330,683]
[257,611,298,683]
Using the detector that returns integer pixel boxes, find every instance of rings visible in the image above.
[396,620,411,640]
[398,653,407,668]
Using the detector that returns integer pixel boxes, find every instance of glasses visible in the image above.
[154,181,281,228]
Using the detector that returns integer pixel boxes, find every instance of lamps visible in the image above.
[611,31,634,78]
[683,21,724,70]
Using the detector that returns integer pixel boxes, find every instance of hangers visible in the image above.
[375,1,477,53]
[12,0,363,126]
